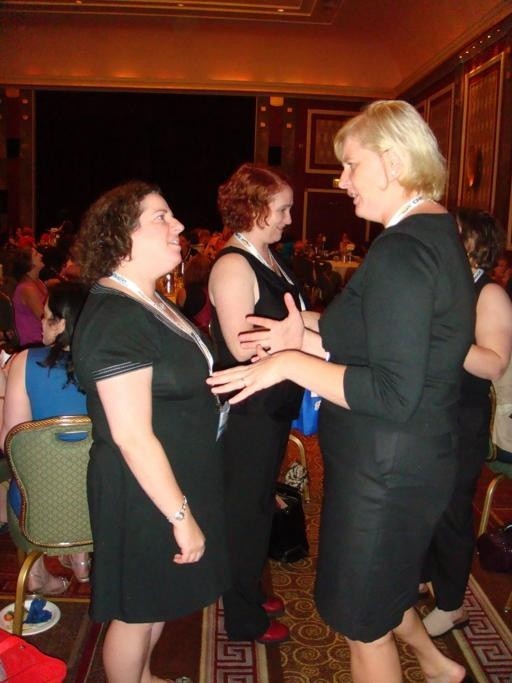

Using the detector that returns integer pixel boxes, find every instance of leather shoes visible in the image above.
[256,593,290,644]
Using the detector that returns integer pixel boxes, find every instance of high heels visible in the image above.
[27,552,92,595]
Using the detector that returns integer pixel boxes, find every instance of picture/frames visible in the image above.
[304,107,362,176]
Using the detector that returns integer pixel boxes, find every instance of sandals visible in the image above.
[422,608,470,638]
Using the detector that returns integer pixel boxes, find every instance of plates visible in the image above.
[1,598,62,637]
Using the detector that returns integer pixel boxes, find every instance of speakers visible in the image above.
[6,138,20,159]
[268,146,282,166]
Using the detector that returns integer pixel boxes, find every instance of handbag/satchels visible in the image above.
[267,483,310,563]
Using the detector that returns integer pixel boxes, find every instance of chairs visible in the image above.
[480,384,512,546]
[3,413,95,639]
[301,258,358,302]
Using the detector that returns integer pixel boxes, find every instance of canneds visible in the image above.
[165,273,173,295]
